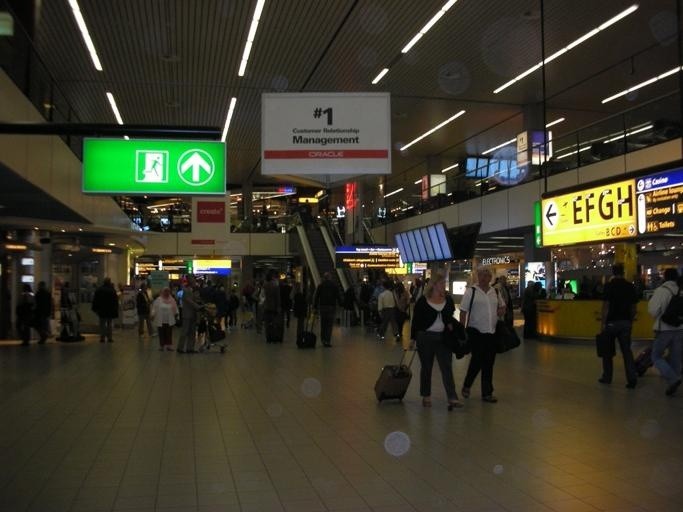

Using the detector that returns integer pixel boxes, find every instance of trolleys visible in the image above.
[195,305,228,354]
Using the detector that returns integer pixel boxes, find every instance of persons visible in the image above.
[597,263,638,390]
[60,281,82,338]
[91,277,119,342]
[337,200,346,233]
[407,275,467,411]
[343,277,430,341]
[243,270,343,347]
[646,267,683,396]
[493,275,542,340]
[16,282,54,345]
[458,268,505,403]
[136,278,239,352]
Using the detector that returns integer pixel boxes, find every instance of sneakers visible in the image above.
[422,399,432,407]
[483,395,497,403]
[599,378,611,384]
[447,399,463,408]
[461,385,469,396]
[626,383,635,389]
[665,380,681,395]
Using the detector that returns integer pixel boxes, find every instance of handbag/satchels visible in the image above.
[596,333,616,357]
[496,321,520,353]
[453,328,479,359]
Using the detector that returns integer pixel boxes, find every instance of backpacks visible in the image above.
[662,284,682,328]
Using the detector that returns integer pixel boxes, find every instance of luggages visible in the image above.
[296,311,316,349]
[374,343,416,403]
[636,347,655,376]
